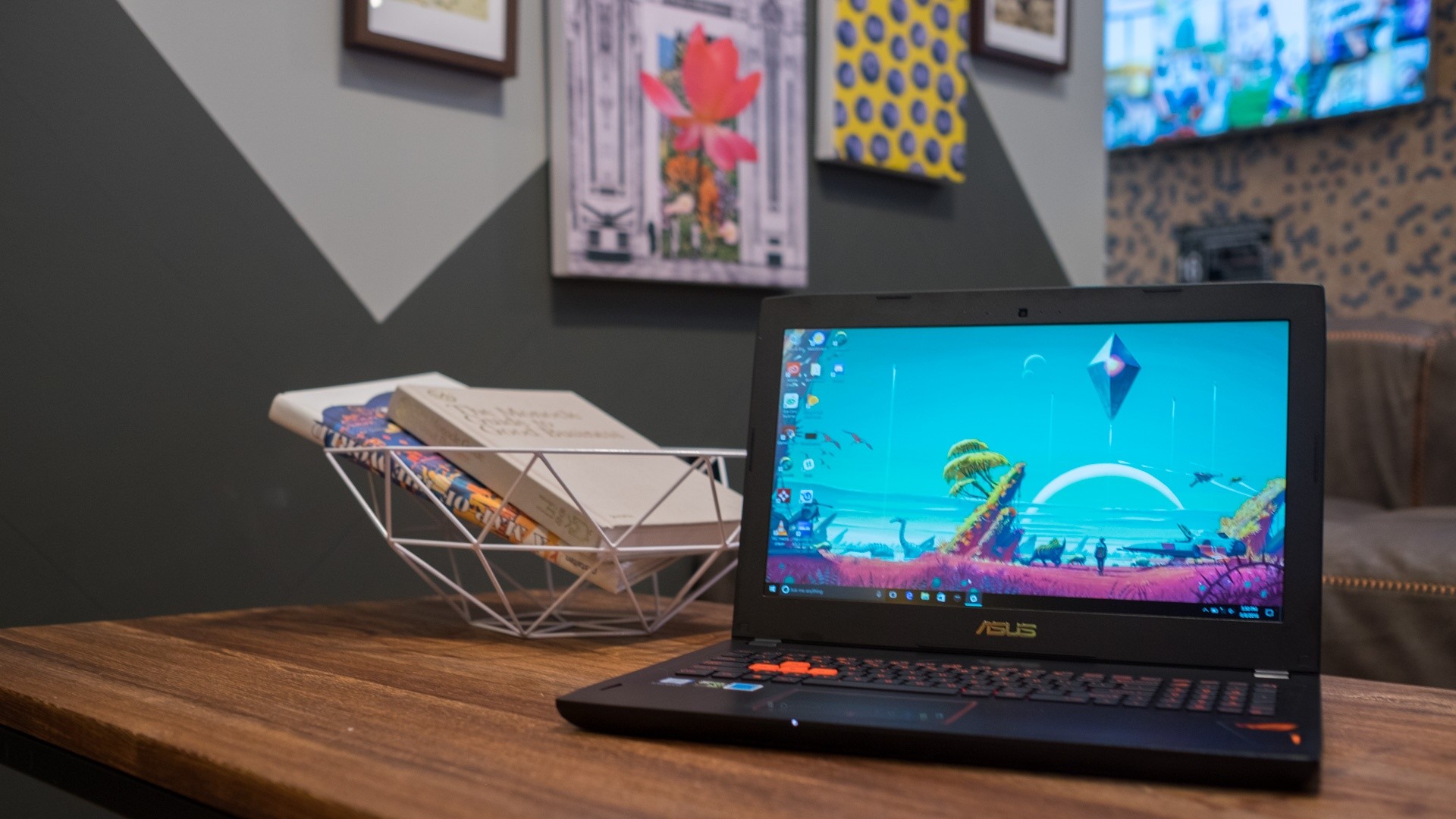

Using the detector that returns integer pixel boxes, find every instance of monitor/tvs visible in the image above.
[1102,0,1436,154]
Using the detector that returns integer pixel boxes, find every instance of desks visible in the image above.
[0,587,1456,819]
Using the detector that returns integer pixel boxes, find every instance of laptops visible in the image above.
[554,280,1326,791]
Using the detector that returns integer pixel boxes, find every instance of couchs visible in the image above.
[1320,319,1456,690]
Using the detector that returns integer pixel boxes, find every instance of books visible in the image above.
[266,372,683,594]
[386,387,743,562]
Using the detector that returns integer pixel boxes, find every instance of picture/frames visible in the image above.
[969,0,1072,74]
[342,0,522,78]
